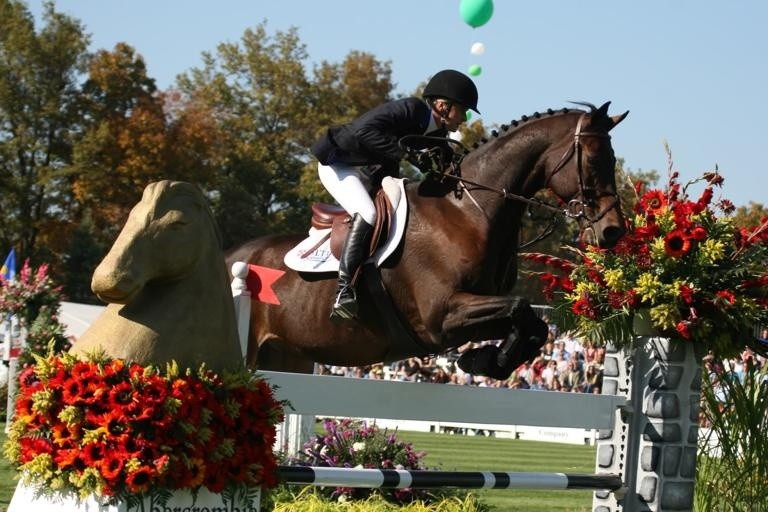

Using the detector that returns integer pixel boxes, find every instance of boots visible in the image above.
[332,211,376,315]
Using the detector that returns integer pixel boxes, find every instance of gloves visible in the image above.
[407,150,433,174]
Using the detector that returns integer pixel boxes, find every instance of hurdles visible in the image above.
[244,334,705,512]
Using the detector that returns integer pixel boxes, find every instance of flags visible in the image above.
[0,249,15,286]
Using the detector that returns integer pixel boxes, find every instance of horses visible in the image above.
[225,101,630,381]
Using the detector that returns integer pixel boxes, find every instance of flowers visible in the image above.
[516,136,768,360]
[3,337,295,512]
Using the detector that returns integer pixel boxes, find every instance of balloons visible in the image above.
[463,110,473,121]
[471,42,485,56]
[469,65,485,77]
[458,0,494,29]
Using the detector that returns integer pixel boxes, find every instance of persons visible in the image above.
[312,70,482,323]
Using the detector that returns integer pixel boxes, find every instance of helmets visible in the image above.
[422,69,482,116]
